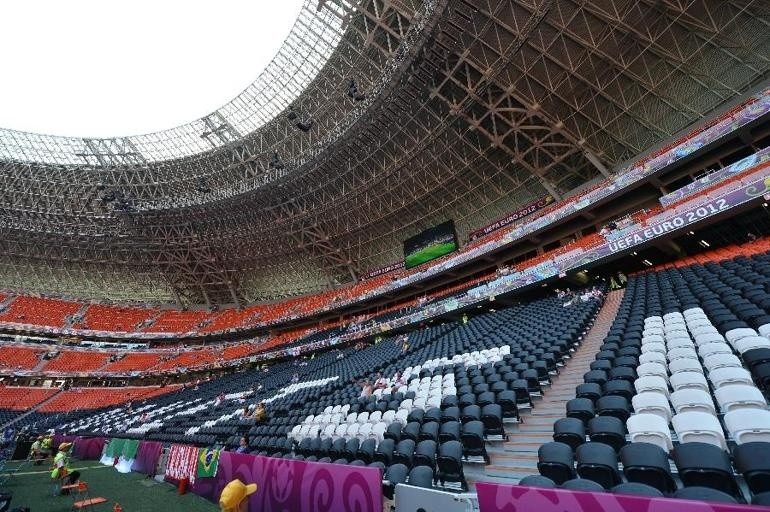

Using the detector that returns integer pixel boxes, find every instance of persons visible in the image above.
[293,347,345,366]
[599,224,612,239]
[243,403,266,422]
[355,335,385,351]
[351,371,407,401]
[462,312,469,324]
[555,270,628,308]
[236,435,251,454]
[139,410,151,424]
[256,383,264,393]
[748,232,765,243]
[395,333,409,354]
[177,374,218,393]
[29,434,80,497]
[414,294,436,310]
[495,264,516,278]
[291,371,299,383]
[125,399,133,415]
[214,391,225,407]
[218,478,257,512]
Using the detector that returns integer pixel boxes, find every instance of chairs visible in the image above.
[148,260,770,507]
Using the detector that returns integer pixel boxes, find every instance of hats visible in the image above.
[219,479,258,509]
[38,433,71,450]
